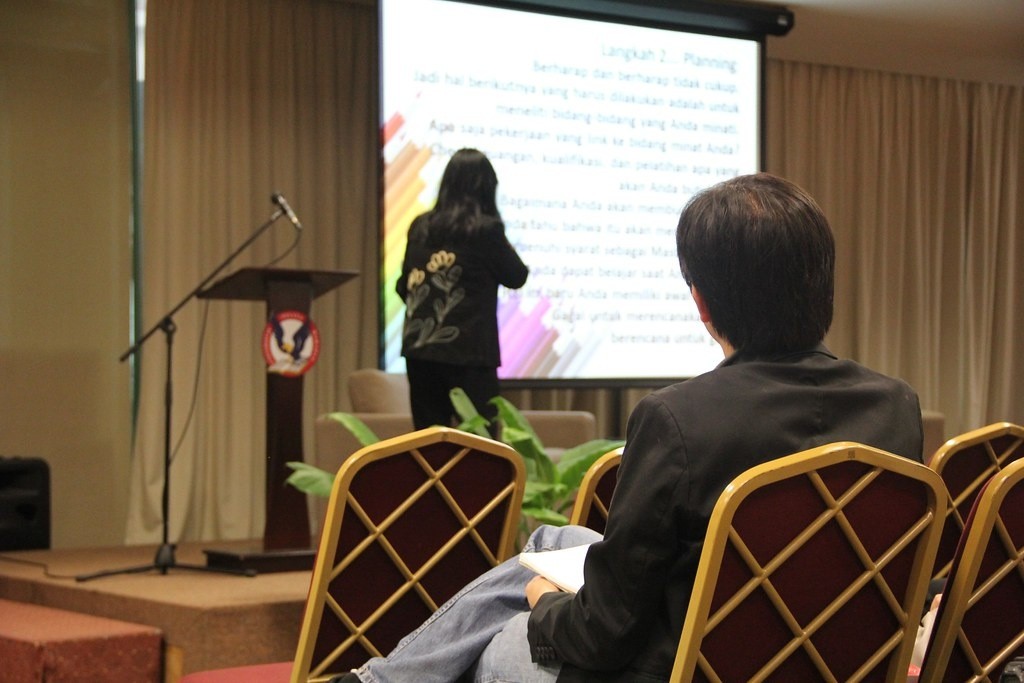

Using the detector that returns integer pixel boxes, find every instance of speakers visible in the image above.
[0,455,51,550]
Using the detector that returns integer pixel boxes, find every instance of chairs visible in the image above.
[571,447,628,537]
[927,420,1024,600]
[178,422,527,682]
[918,456,1024,683]
[669,441,948,683]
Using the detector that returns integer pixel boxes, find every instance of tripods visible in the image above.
[75,208,287,581]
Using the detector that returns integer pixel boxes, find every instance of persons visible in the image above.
[326,173,922,683]
[396,148,528,440]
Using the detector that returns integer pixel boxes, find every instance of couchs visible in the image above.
[313,367,597,565]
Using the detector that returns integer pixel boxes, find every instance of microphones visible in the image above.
[270,191,303,231]
